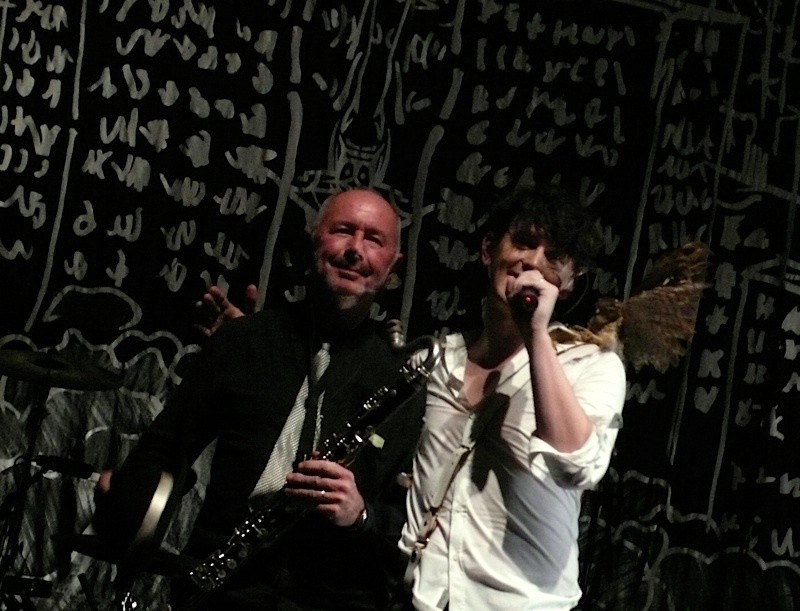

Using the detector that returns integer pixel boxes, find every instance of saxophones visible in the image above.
[183,319,447,599]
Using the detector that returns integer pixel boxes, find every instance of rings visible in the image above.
[318,489,326,500]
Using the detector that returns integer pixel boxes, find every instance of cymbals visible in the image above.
[0,349,124,392]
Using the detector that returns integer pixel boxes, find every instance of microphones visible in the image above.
[515,287,538,311]
[34,455,94,479]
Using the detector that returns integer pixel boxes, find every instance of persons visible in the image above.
[94,188,426,611]
[190,192,627,611]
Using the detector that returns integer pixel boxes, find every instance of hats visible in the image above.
[91,460,197,577]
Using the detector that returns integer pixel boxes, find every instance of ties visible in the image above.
[250,343,331,496]
